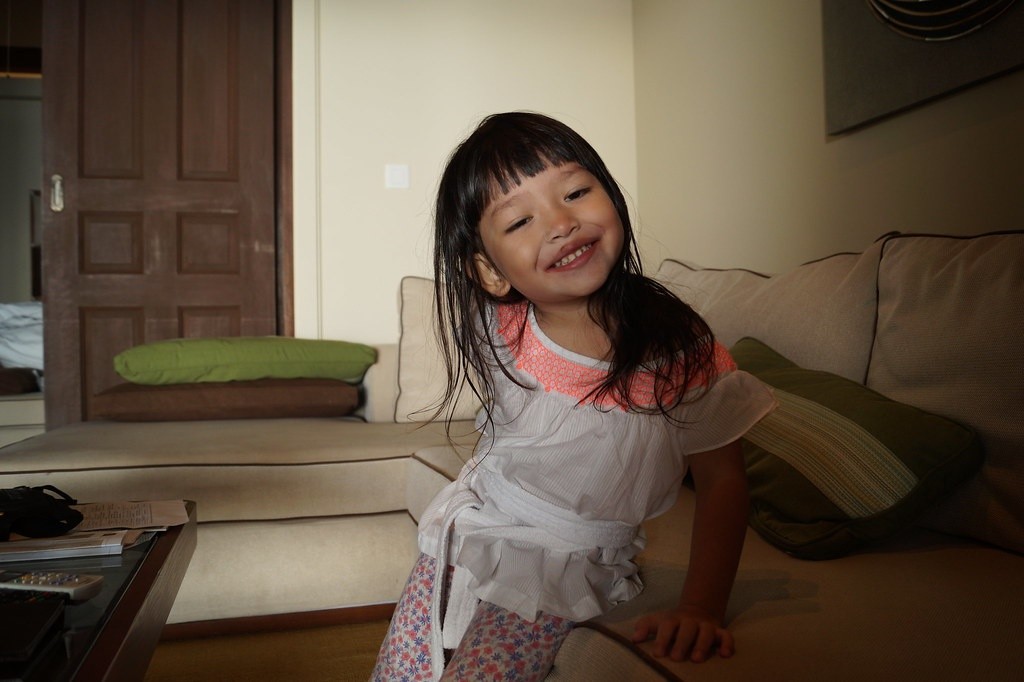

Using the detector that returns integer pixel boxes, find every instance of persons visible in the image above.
[361,108,779,682]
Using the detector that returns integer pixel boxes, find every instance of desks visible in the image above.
[0,497,199,682]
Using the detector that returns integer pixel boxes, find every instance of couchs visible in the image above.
[0,228,1024,682]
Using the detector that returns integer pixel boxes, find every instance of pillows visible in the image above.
[110,335,378,387]
[865,229,1024,558]
[394,275,490,424]
[86,378,368,418]
[688,334,984,567]
[651,230,904,389]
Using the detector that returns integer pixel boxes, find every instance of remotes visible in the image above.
[0,570,105,604]
